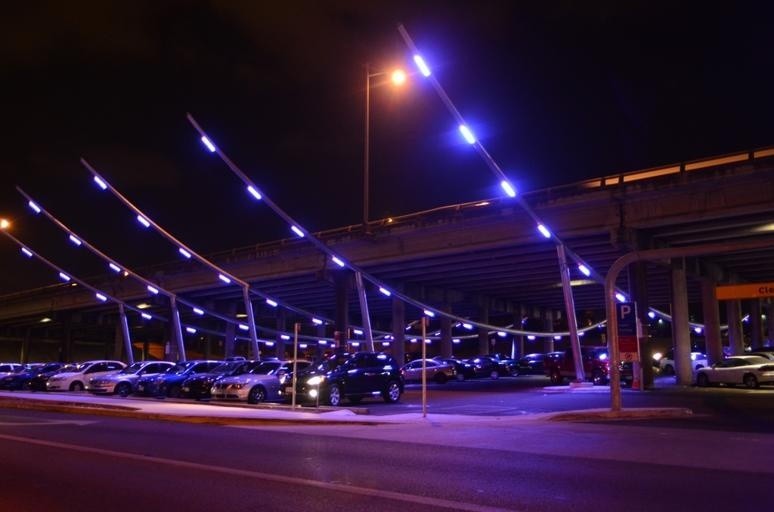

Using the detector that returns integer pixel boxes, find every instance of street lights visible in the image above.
[360,56,410,216]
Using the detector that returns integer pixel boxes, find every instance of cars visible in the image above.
[210,358,313,403]
[46,358,129,391]
[400,347,635,388]
[0,359,81,392]
[658,344,773,389]
[181,359,245,400]
[87,361,178,398]
[134,360,226,399]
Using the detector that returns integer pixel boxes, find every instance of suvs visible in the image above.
[279,352,407,406]
